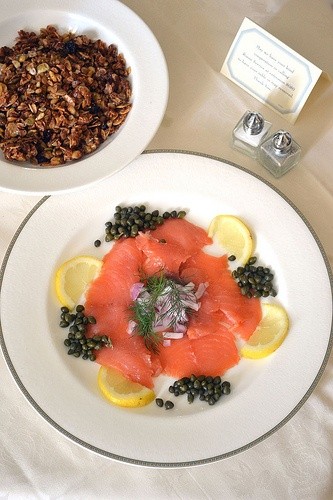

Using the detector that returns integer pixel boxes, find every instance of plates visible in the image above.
[1,0,170,194]
[0,147,333,469]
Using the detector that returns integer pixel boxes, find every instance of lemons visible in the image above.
[240,304,290,358]
[208,214,254,267]
[97,365,156,409]
[55,255,103,311]
